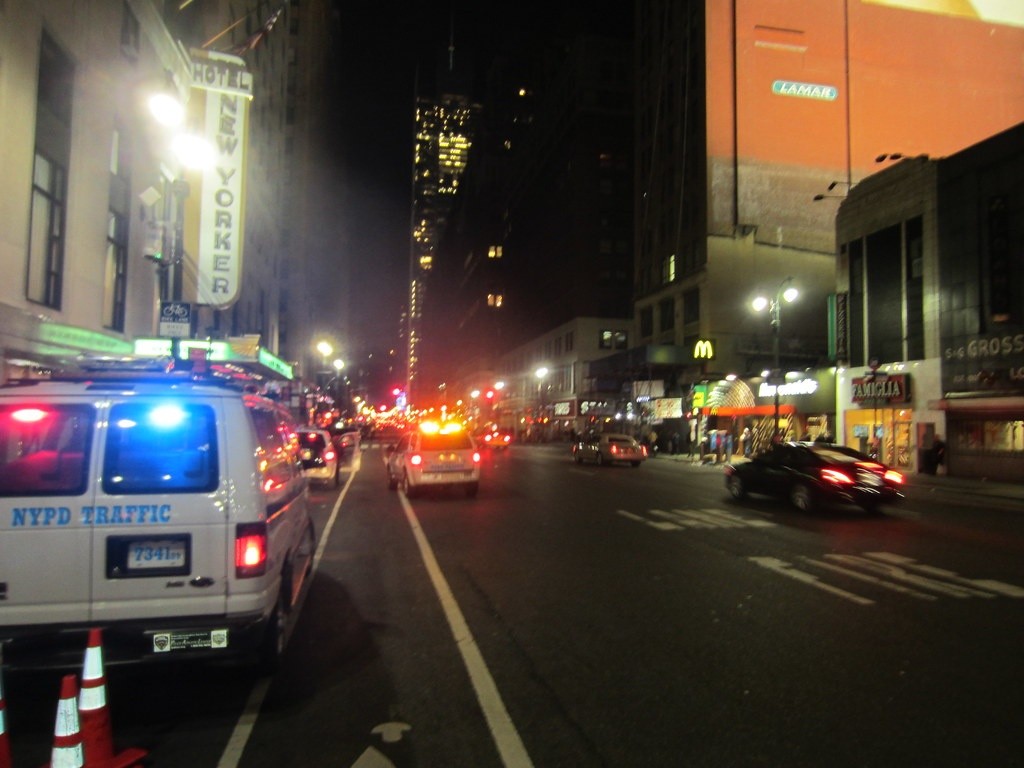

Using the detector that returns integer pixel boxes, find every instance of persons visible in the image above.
[930,433,945,474]
[743,431,752,457]
[670,432,679,453]
[716,434,731,464]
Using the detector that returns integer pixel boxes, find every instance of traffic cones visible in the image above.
[50,673,85,768]
[0,691,14,767]
[79,628,144,768]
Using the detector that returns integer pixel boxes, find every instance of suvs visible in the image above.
[386,429,480,500]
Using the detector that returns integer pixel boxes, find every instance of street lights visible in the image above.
[751,275,800,445]
[144,90,220,366]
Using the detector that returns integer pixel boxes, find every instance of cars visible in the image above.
[573,433,648,468]
[333,427,361,448]
[477,431,511,448]
[295,428,339,489]
[723,441,905,517]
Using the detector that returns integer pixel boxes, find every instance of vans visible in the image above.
[0,365,316,677]
